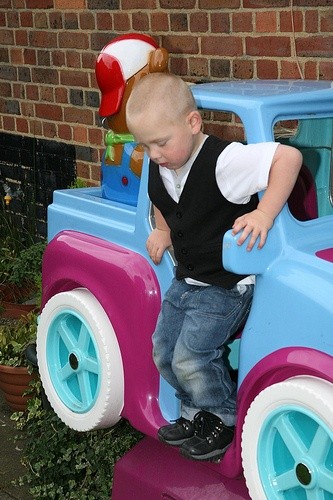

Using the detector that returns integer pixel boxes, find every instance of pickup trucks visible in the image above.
[34,79,333,500]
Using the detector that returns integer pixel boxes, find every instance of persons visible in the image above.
[126,73,303,462]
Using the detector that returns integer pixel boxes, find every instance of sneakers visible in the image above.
[181,409,236,461]
[157,417,196,446]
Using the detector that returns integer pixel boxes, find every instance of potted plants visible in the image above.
[0,317,37,417]
